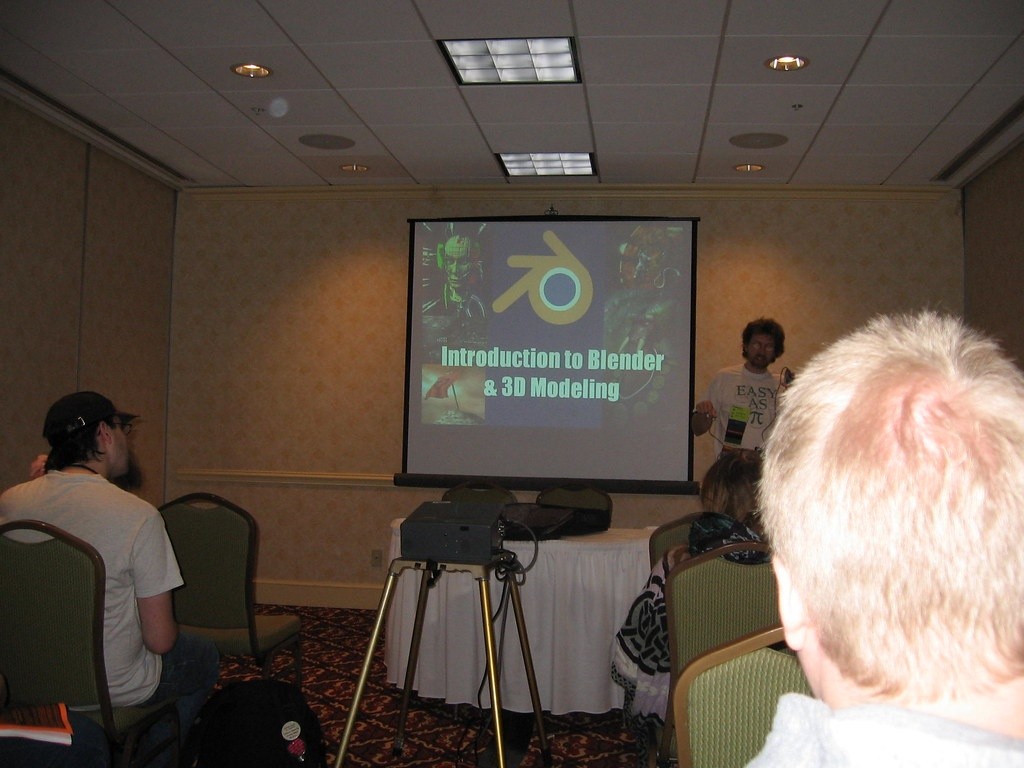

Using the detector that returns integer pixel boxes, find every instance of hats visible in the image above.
[40,390,140,445]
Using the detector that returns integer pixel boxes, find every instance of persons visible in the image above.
[610,311,1024,768]
[0,389,224,768]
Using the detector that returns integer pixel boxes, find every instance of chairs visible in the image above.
[648,513,815,768]
[0,518,187,768]
[156,492,303,688]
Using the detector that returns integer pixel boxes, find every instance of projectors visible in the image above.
[401,500,506,564]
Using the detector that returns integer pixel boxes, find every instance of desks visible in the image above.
[384,518,660,717]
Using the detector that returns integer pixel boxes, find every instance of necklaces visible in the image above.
[67,462,98,475]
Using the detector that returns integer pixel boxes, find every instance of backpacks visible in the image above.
[177,678,328,768]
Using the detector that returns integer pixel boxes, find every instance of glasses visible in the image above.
[113,423,133,435]
